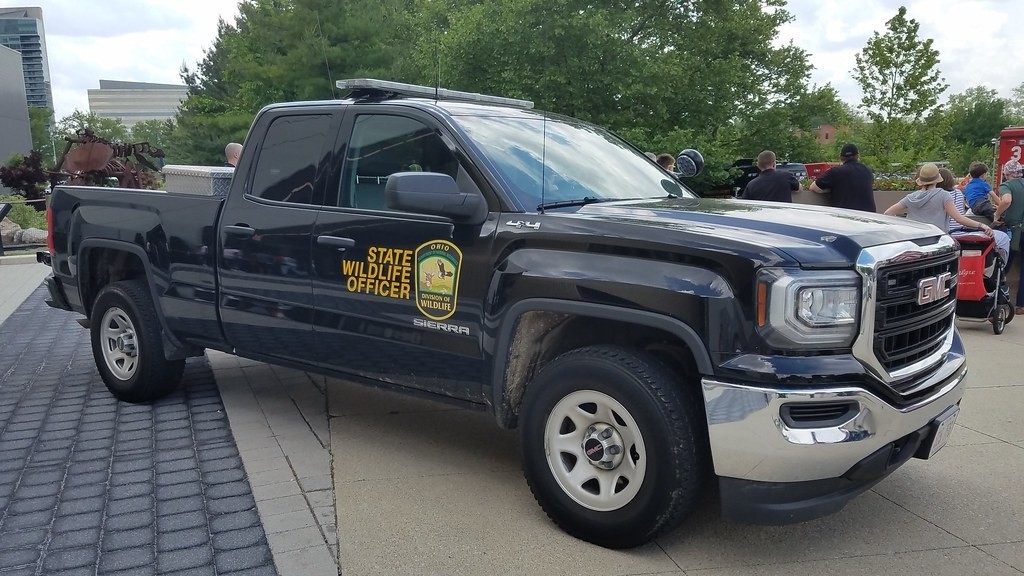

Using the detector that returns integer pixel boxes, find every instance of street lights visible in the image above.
[52,134,59,165]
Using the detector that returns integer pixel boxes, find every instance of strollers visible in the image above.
[947,226,1015,336]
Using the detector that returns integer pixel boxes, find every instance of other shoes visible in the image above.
[1014,306,1024,315]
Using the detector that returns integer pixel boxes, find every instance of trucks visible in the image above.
[991,124,1024,207]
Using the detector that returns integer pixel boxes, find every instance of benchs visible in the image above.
[162,163,235,198]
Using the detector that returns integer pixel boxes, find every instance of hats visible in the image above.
[916,163,944,187]
[1004,159,1024,177]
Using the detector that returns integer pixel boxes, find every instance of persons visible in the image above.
[644,152,676,174]
[809,144,877,213]
[884,160,1024,315]
[741,150,803,203]
[223,143,244,168]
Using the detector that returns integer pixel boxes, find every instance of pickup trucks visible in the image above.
[35,79,965,553]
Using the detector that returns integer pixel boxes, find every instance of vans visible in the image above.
[728,161,806,188]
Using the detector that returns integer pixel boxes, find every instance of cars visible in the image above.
[45,185,51,194]
